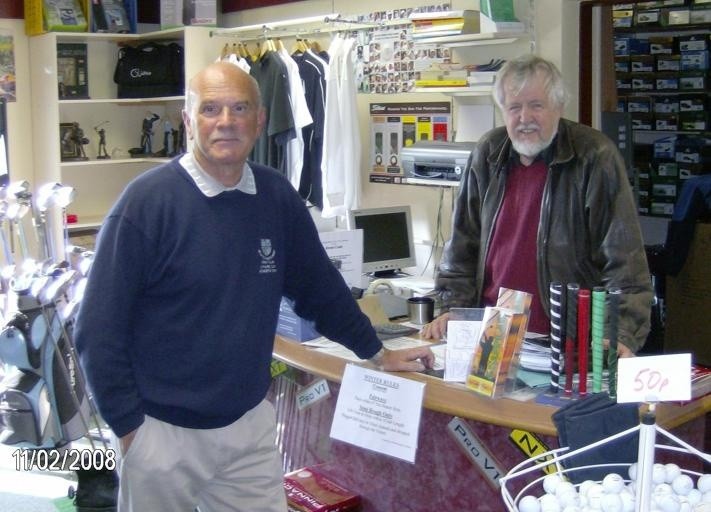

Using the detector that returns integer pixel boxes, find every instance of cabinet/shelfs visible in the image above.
[615,25,711,246]
[406,31,533,190]
[30,26,227,230]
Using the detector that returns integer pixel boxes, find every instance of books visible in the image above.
[405,0,518,88]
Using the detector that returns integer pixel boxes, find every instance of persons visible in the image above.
[67,62,437,512]
[418,57,657,367]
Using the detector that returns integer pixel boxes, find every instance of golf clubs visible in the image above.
[0,180,110,453]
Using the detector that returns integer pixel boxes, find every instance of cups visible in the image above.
[404,297,434,325]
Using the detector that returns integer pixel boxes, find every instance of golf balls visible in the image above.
[518,463,711,512]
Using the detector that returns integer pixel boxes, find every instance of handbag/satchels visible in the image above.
[113,41,184,99]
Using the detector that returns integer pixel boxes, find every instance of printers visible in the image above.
[399,139,481,183]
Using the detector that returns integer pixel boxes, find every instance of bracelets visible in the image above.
[371,346,387,365]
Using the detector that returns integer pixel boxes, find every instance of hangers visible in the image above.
[219,38,322,62]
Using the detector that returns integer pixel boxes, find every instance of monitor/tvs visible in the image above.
[346,205,417,275]
